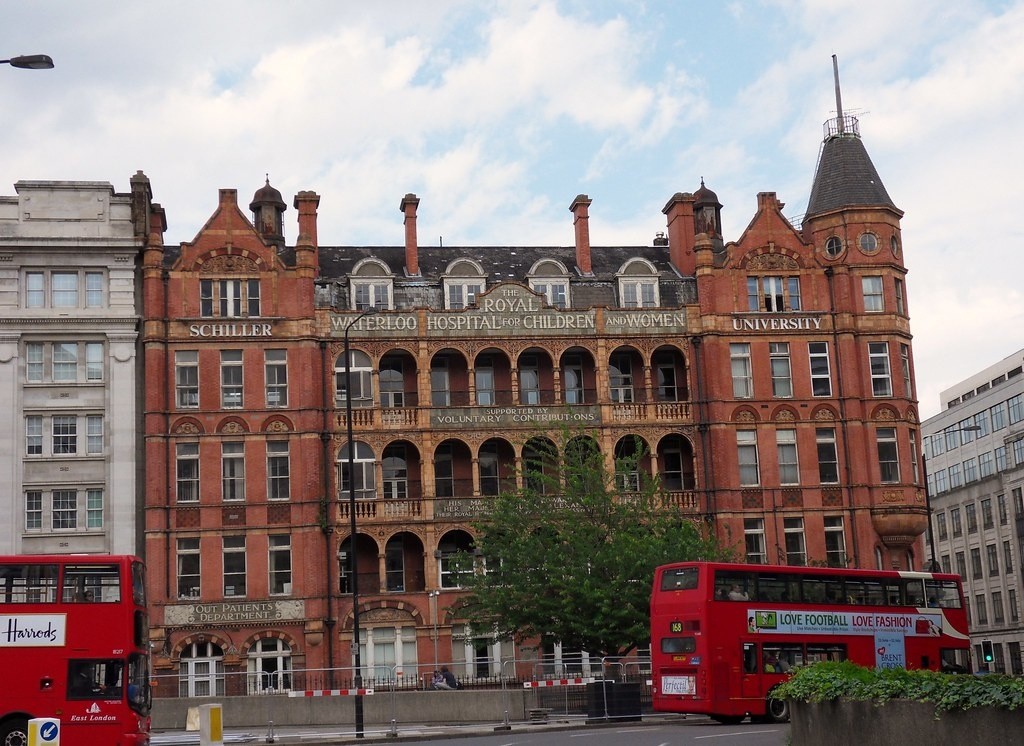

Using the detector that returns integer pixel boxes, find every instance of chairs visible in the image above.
[774,660,783,673]
[750,589,942,609]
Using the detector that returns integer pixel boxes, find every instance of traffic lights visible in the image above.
[981,639,995,663]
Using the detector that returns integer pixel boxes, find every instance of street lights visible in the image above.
[344,306,380,739]
[922,424,982,573]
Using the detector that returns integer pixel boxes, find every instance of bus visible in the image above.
[650,560,973,724]
[0,552,153,746]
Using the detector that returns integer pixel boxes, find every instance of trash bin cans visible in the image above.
[587,682,642,721]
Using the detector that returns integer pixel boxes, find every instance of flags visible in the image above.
[777,547,785,560]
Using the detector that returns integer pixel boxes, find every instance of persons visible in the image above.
[942,657,959,668]
[715,583,939,608]
[688,676,694,694]
[84,590,94,602]
[763,652,793,672]
[748,617,760,633]
[432,665,458,690]
[68,658,107,697]
